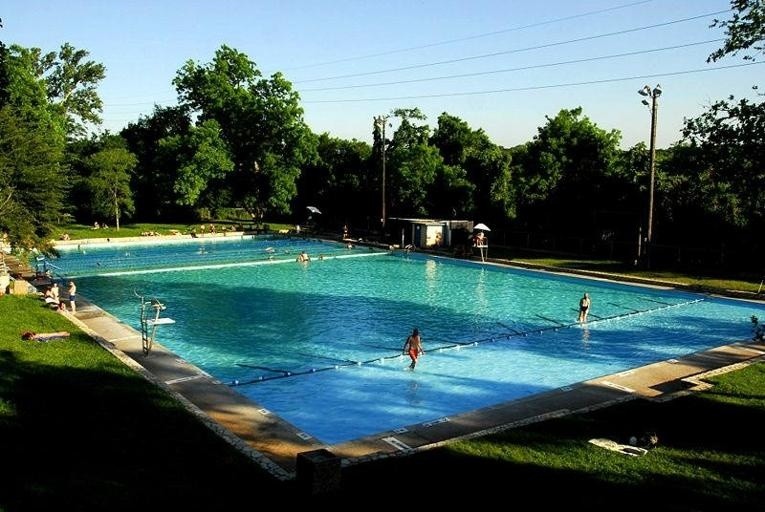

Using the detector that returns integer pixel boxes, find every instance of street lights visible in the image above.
[635,79,664,268]
[371,111,395,232]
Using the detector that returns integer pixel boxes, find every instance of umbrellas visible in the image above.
[473,223,491,232]
[306,206,322,214]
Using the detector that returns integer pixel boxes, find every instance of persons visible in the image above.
[403,329,424,369]
[199,223,236,236]
[579,293,590,321]
[306,216,316,226]
[343,225,347,239]
[44,281,77,314]
[299,251,309,267]
[22,332,70,339]
[473,230,484,248]
